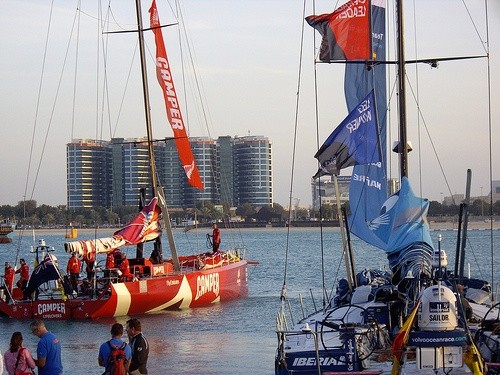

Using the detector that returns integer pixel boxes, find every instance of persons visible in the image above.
[67,248,131,298]
[209,223,221,253]
[4,259,29,304]
[98,318,149,375]
[0,320,63,375]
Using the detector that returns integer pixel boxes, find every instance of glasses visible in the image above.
[31,328,38,334]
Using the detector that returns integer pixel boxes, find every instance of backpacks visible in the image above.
[107,341,129,375]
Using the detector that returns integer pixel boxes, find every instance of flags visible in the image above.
[304,0,374,62]
[312,88,383,179]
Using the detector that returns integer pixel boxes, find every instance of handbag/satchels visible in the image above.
[13,347,36,375]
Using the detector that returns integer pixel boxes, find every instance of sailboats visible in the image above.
[274,0,500,375]
[1,0,263,324]
